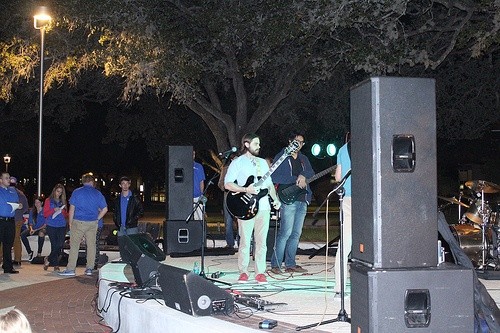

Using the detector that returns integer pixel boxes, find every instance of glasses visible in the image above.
[294,138,305,146]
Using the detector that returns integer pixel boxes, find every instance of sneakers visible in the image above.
[85,269,92,275]
[57,270,76,275]
[238,273,268,284]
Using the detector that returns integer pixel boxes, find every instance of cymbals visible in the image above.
[437,196,470,208]
[464,180,499,194]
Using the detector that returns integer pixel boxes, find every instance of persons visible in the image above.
[43,183,68,272]
[0,172,21,274]
[112,176,143,236]
[218,152,241,250]
[335,142,352,298]
[20,197,47,261]
[0,309,32,333]
[92,218,103,272]
[265,158,273,169]
[192,150,206,220]
[0,176,28,268]
[224,132,280,285]
[270,131,315,273]
[57,172,108,276]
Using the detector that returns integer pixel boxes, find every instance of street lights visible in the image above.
[4,154,11,173]
[139,184,144,202]
[33,4,52,197]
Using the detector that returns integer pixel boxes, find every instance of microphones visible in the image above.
[218,147,237,156]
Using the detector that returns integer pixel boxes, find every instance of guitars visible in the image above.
[225,139,300,221]
[277,163,338,206]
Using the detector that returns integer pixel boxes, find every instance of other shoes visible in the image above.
[28,251,41,261]
[43,263,60,271]
[12,261,21,269]
[271,265,307,274]
[4,270,19,274]
[333,293,349,301]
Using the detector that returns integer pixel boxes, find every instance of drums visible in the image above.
[466,198,491,223]
[460,212,482,227]
[449,223,483,267]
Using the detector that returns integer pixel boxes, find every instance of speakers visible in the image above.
[160,263,235,317]
[348,75,473,333]
[118,221,167,288]
[163,145,206,256]
